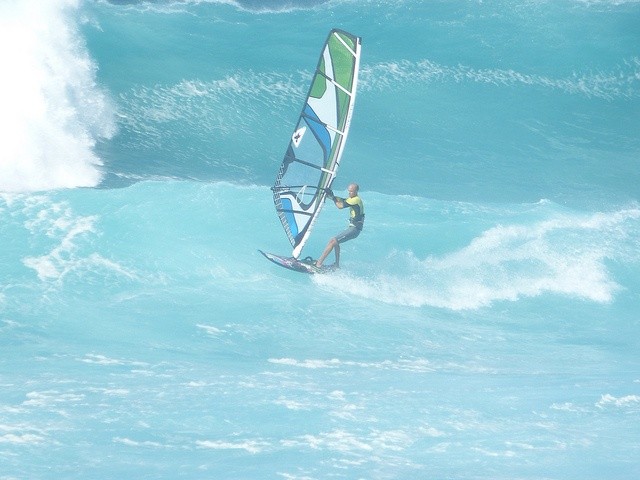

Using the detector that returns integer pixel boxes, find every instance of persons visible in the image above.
[316,184,364,268]
